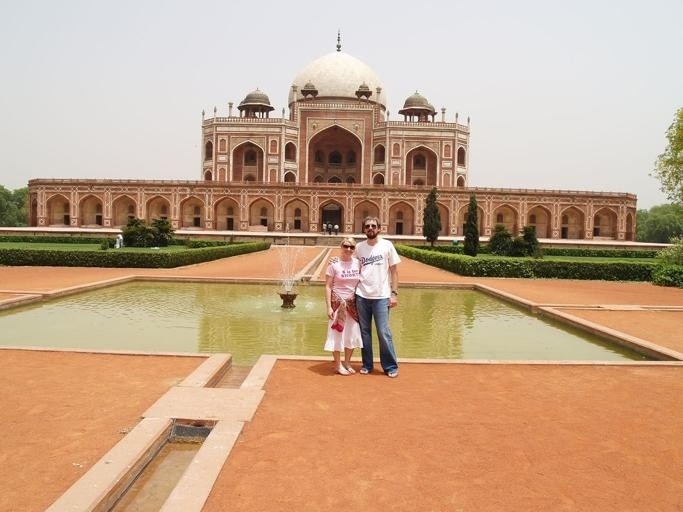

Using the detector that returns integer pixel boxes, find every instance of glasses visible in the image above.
[365,224,377,229]
[343,245,356,251]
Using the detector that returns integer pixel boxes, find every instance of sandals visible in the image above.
[336,368,368,375]
[388,371,397,378]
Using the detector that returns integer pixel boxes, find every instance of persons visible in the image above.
[323,216,401,377]
